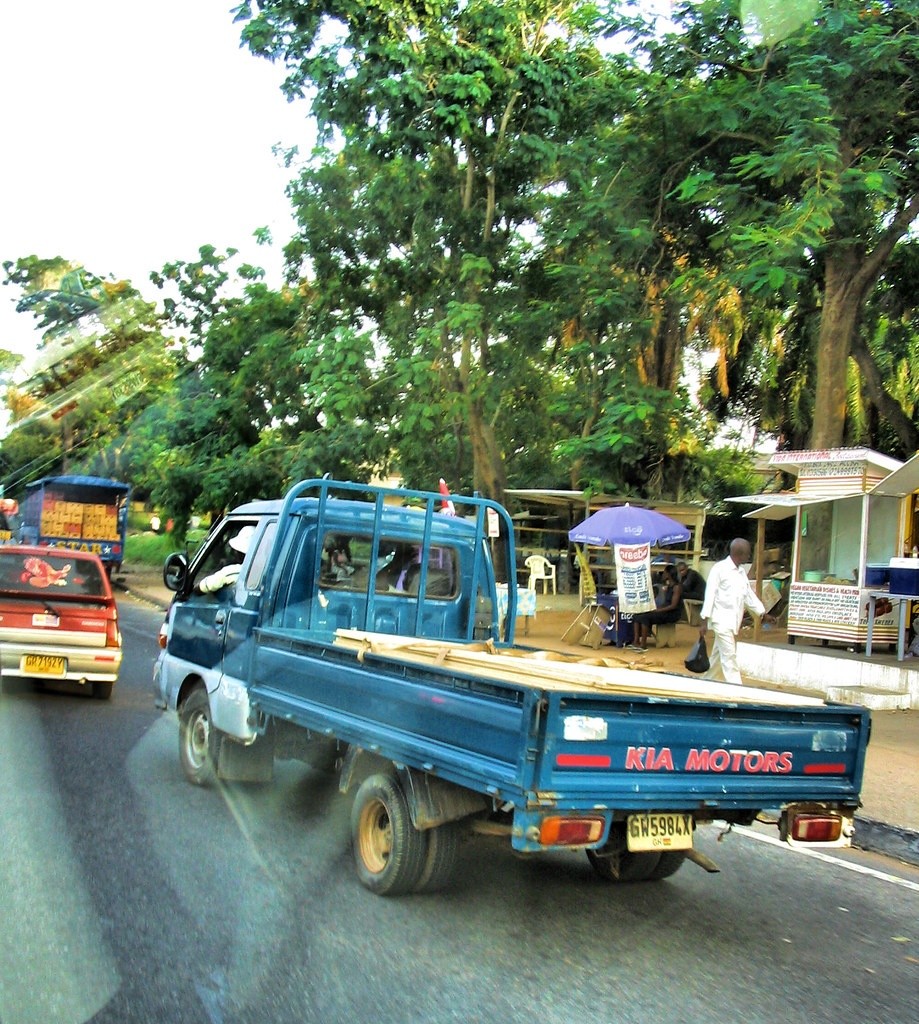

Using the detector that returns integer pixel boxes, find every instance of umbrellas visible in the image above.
[568,504,691,548]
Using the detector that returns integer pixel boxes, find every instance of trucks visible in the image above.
[17,475,130,580]
[152,473,872,896]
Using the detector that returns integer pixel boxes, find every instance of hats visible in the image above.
[229,525,256,554]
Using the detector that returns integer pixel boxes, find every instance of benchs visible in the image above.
[682,598,703,626]
[650,622,676,648]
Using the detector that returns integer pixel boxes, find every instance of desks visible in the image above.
[587,562,668,588]
[786,581,907,653]
[585,595,636,647]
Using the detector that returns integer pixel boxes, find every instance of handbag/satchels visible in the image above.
[685,637,711,673]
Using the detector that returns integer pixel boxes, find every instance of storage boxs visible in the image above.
[745,570,791,616]
[42,490,120,541]
[866,557,919,596]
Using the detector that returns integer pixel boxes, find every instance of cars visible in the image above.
[0,545,123,699]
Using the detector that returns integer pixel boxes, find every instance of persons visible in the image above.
[149,514,162,533]
[700,537,778,685]
[166,574,183,589]
[622,562,707,653]
[187,525,420,597]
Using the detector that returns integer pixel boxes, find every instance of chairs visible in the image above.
[524,555,556,595]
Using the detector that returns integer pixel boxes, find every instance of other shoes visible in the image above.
[623,645,649,653]
